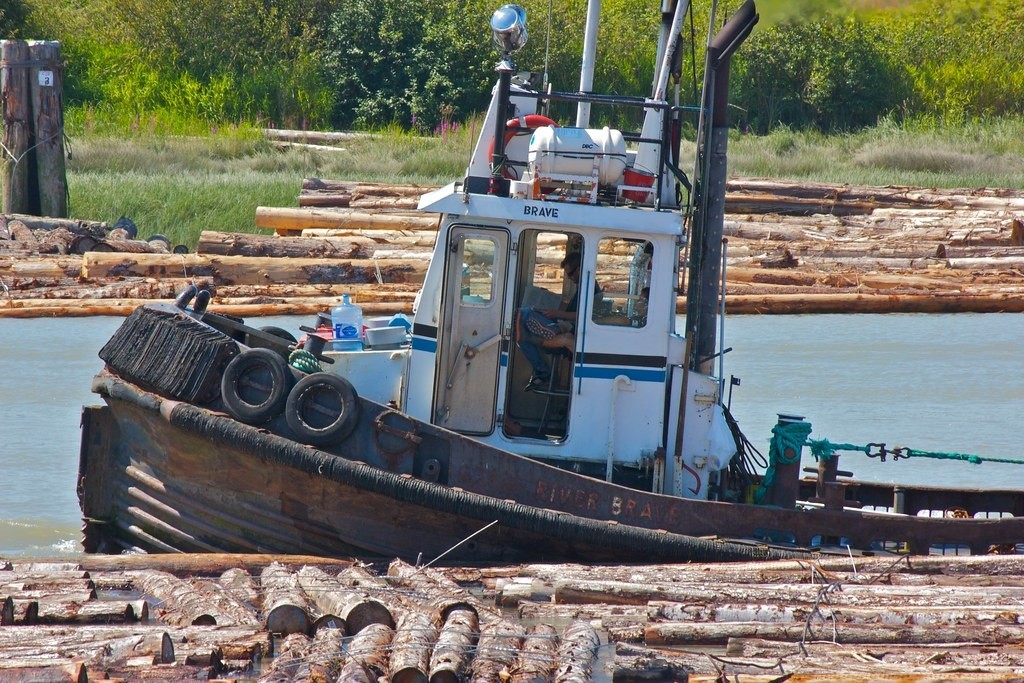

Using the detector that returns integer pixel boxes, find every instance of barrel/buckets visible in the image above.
[621,162,655,203]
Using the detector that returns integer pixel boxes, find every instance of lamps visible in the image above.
[490,4,530,60]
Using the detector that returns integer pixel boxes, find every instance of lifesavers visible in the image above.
[490,115,557,195]
[286,372,359,450]
[223,348,291,426]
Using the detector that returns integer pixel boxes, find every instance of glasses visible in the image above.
[567,267,577,276]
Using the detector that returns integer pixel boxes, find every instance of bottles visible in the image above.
[332,294,363,351]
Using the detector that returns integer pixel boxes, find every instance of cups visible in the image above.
[510,180,531,198]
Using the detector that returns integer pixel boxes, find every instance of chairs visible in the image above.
[527,347,575,434]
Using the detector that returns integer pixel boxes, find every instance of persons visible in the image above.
[514,254,604,393]
[515,287,651,354]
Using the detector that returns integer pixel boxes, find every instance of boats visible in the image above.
[74,2,1024,567]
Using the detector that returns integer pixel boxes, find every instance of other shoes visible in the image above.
[526,318,560,341]
[523,376,548,391]
[514,309,531,343]
